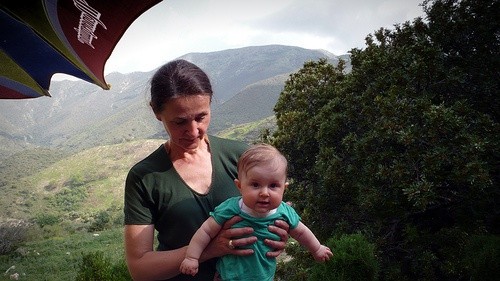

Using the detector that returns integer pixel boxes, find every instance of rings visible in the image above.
[228,240,234,250]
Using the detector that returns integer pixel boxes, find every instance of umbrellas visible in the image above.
[0,1,162,101]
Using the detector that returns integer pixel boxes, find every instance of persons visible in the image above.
[124,59,265,281]
[179,143,333,281]
